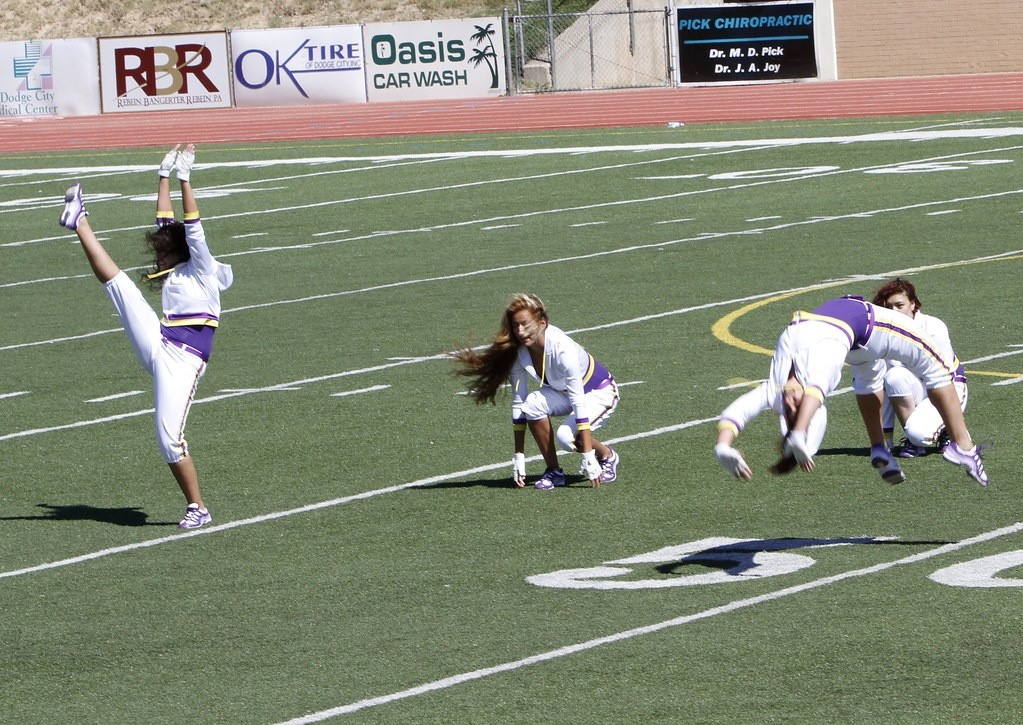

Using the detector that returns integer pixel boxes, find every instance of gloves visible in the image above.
[158,144,181,178]
[512,452,526,488]
[176,143,195,182]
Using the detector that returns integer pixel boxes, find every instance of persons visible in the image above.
[59,141,234,527]
[873,278,968,458]
[445,294,621,491]
[714,295,989,488]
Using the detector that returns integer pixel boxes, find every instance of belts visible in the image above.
[593,377,613,389]
[168,339,207,361]
[841,294,875,351]
[954,377,966,383]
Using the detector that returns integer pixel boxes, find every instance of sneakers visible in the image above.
[869,441,906,485]
[59,182,88,230]
[179,503,212,529]
[943,440,988,487]
[935,434,950,453]
[535,467,565,489]
[898,435,926,458]
[600,446,619,482]
